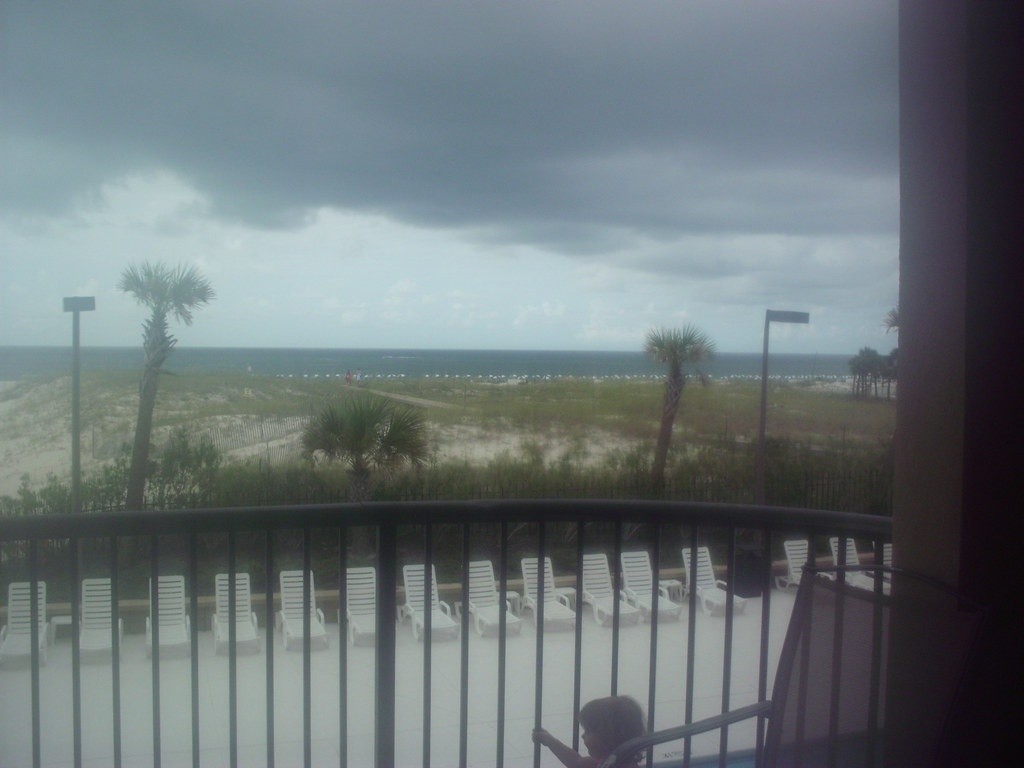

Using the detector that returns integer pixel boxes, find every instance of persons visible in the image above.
[532,694,647,768]
[346,368,362,385]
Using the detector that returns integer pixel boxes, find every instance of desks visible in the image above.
[554,586,576,608]
[47,614,73,645]
[658,578,681,603]
[497,590,522,616]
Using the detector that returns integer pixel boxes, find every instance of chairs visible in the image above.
[829,536,890,594]
[871,540,892,581]
[583,554,639,625]
[774,540,833,594]
[280,569,326,650]
[144,575,191,658]
[600,540,991,768]
[520,557,576,631]
[337,566,376,646]
[78,576,123,660]
[0,581,50,664]
[621,550,680,621]
[397,563,459,640]
[682,546,747,615]
[455,560,523,636]
[211,572,257,653]
